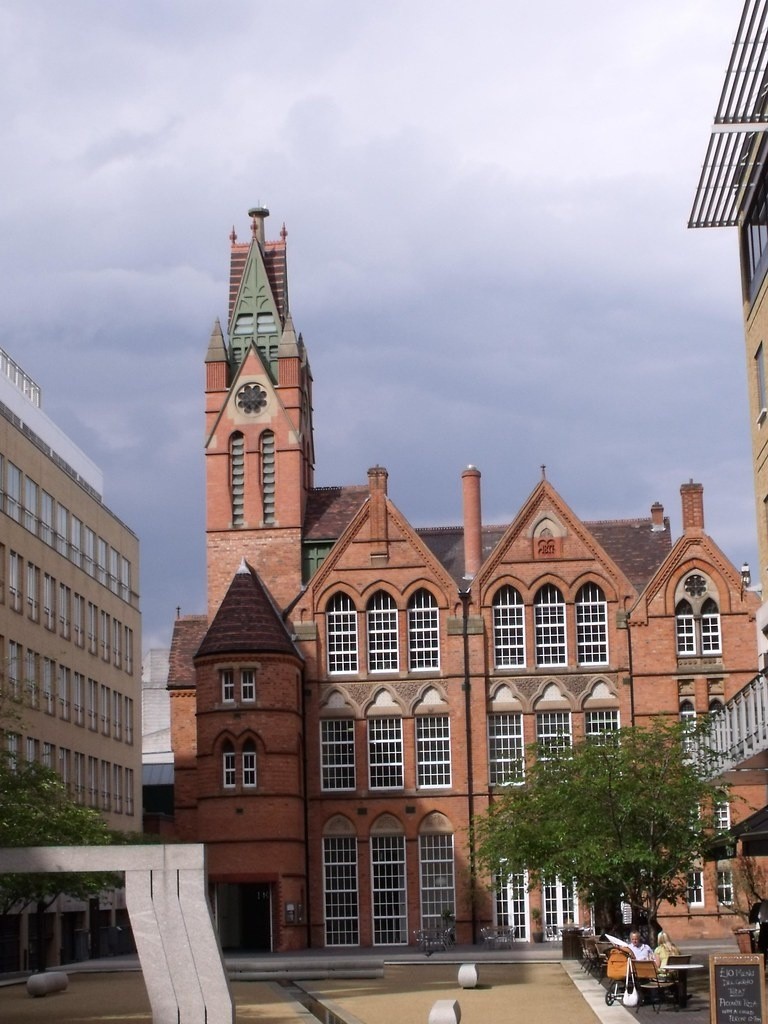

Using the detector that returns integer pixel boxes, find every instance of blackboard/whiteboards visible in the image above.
[708,953,766,1024]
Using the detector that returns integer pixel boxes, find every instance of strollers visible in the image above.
[604,945,635,1004]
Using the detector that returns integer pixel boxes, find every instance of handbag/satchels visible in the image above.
[622,986,638,1006]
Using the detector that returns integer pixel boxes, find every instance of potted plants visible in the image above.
[530,907,544,943]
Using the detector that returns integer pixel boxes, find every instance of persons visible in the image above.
[647,930,681,983]
[628,928,656,961]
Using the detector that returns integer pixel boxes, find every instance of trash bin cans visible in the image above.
[562,925,584,961]
[72,927,92,962]
[120,925,137,953]
[100,926,122,957]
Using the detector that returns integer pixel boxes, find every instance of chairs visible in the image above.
[479,926,517,952]
[631,959,679,1014]
[413,928,455,952]
[579,936,691,991]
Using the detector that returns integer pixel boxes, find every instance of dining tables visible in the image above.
[488,926,510,949]
[422,929,445,951]
[663,964,704,1012]
